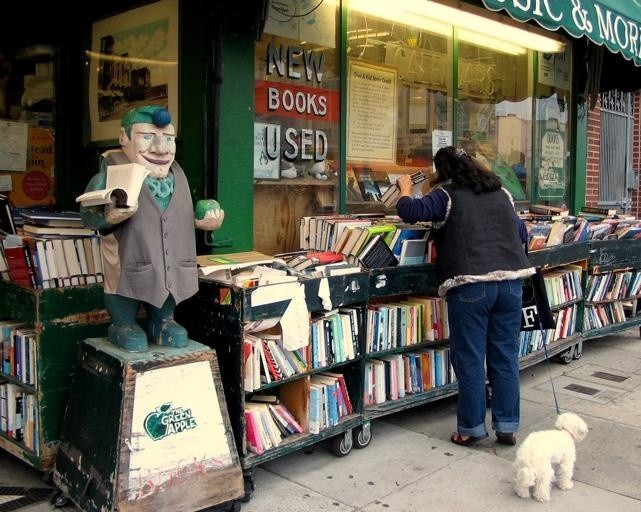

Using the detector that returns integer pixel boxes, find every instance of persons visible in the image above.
[79,104,226,355]
[396,146,539,448]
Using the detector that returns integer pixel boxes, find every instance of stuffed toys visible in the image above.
[282,164,297,178]
[307,160,328,180]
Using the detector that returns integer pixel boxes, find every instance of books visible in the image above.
[76,162,151,208]
[0,194,105,454]
[517,205,641,358]
[197,168,457,455]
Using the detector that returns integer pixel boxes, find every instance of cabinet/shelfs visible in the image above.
[0,280,146,473]
[353,268,486,422]
[175,271,373,471]
[518,243,584,369]
[584,240,641,341]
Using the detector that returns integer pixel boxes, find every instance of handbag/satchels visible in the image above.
[521,267,555,331]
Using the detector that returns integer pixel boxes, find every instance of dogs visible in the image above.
[512,411,589,503]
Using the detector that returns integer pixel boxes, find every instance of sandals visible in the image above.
[496,431,516,445]
[452,432,489,446]
